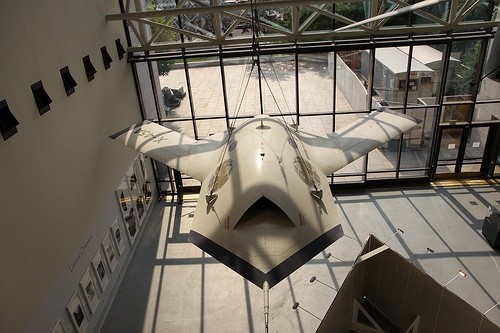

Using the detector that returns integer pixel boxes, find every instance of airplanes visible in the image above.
[107,106,423,332]
